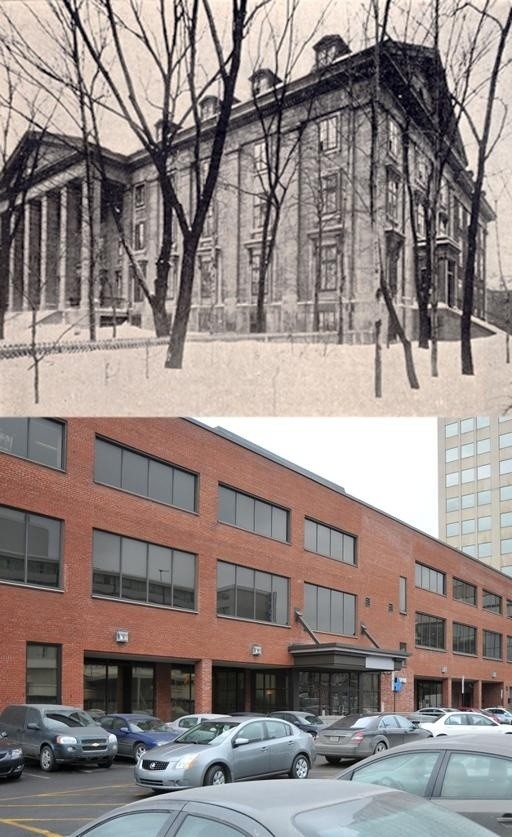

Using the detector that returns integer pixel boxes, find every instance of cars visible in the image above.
[161,713,232,740]
[267,711,332,740]
[133,716,316,796]
[70,778,501,836]
[95,713,184,763]
[227,712,268,717]
[1,730,26,782]
[313,713,433,766]
[333,736,512,836]
[412,704,512,738]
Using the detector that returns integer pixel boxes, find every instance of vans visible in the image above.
[2,705,119,771]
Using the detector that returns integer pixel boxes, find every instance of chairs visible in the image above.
[489,759,507,782]
[444,761,480,796]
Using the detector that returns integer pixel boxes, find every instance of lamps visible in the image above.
[117,631,129,642]
[252,645,262,656]
